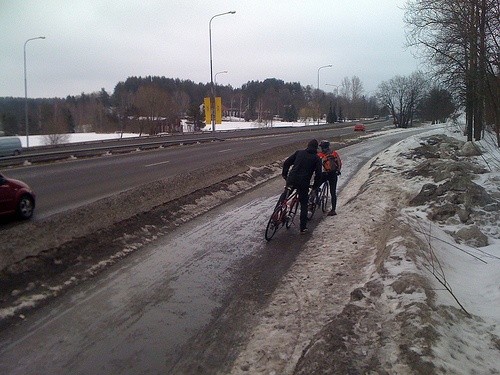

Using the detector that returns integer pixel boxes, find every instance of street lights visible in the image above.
[24,36,46,148]
[209,11,236,131]
[317,65,333,125]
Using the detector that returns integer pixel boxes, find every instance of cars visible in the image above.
[0,172,35,220]
[353,123,366,131]
[0,136,23,157]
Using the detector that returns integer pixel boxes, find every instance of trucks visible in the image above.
[374,115,379,120]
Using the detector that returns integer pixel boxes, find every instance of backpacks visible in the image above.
[319,152,338,171]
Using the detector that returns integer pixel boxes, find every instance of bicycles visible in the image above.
[265,171,341,241]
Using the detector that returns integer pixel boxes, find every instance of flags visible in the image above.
[204,98,211,124]
[215,96,222,124]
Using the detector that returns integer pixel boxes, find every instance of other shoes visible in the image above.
[301,228,309,234]
[327,210,337,216]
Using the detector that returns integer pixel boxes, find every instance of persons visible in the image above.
[282,139,322,233]
[310,140,342,216]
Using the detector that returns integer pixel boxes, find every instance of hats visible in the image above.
[307,139,318,147]
[319,140,330,147]
